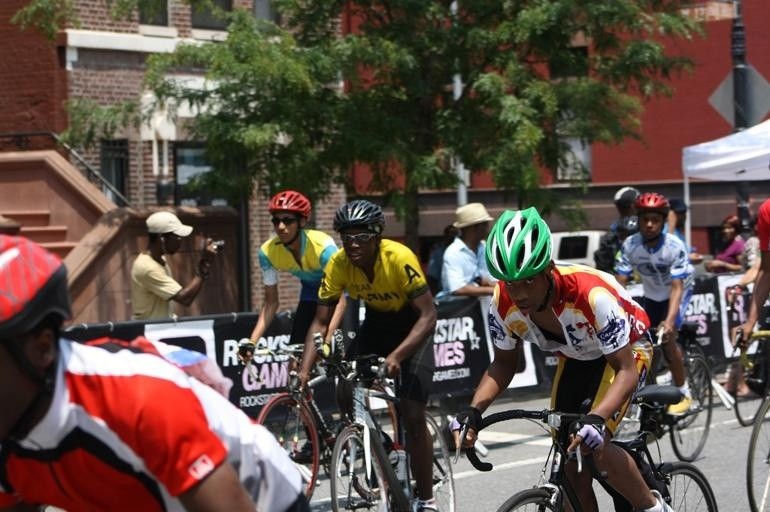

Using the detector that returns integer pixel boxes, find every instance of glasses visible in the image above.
[270,216,299,226]
[340,234,378,243]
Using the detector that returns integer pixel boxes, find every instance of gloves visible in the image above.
[568,414,606,451]
[449,408,481,435]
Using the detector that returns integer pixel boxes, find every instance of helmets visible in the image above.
[636,193,670,216]
[0,234,70,332]
[485,206,550,280]
[268,192,311,219]
[333,200,385,232]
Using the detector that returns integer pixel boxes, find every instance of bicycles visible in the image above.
[616,274,770,512]
[466,385,719,512]
[234,340,402,503]
[305,333,457,512]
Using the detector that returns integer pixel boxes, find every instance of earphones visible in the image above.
[160,236,165,244]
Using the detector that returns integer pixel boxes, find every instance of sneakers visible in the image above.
[666,395,692,416]
[290,437,323,464]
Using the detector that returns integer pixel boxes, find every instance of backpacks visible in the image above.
[595,226,629,270]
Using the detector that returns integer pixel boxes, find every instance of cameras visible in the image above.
[199,239,226,259]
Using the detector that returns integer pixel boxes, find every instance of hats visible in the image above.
[616,188,638,207]
[147,212,193,238]
[453,202,494,228]
[668,197,688,213]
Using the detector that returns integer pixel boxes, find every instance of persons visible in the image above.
[704,199,770,463]
[125,211,221,327]
[421,202,499,451]
[447,207,674,512]
[594,186,704,416]
[234,191,437,512]
[1,233,313,509]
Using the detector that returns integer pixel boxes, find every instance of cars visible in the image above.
[550,229,606,268]
